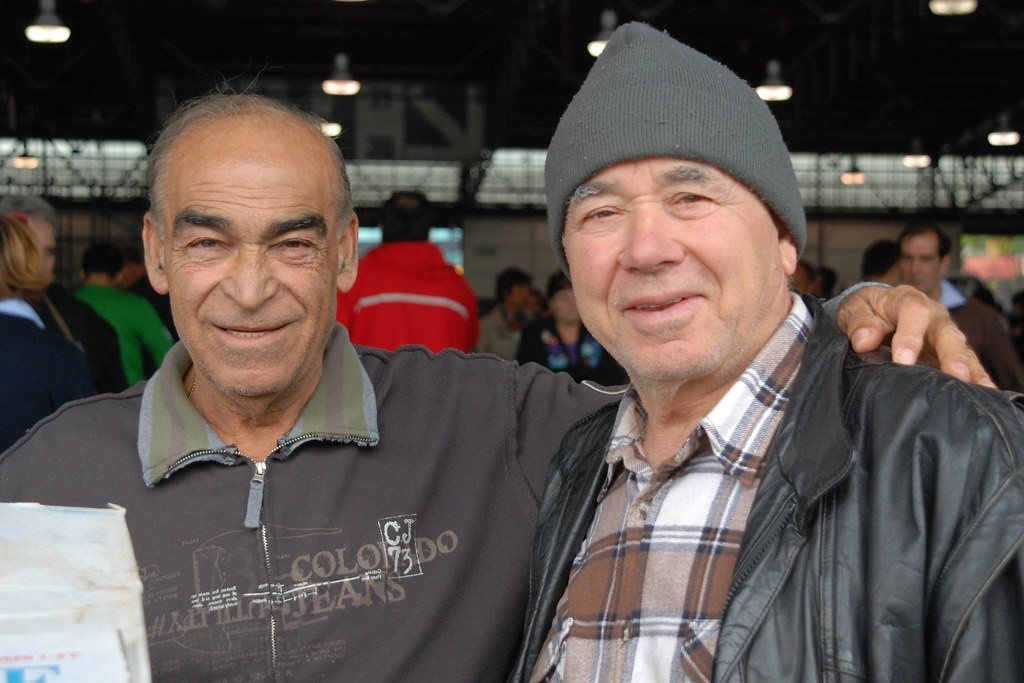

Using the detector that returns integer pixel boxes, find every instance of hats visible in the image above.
[544,20,808,283]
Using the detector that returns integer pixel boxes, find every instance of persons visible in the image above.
[479,264,627,384]
[336,188,482,358]
[795,221,1024,407]
[0,85,997,681]
[506,21,1024,682]
[0,201,176,454]
